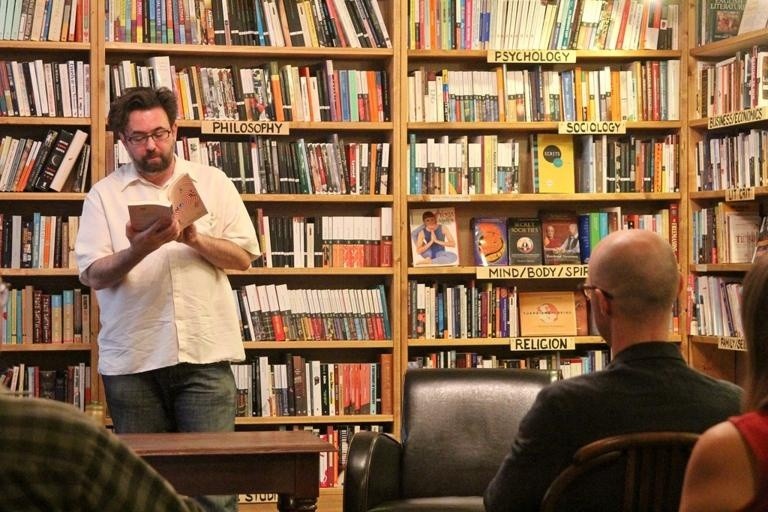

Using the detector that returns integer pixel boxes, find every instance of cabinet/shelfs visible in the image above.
[402,1,687,413]
[686,0,768,391]
[99,0,401,496]
[0,0,99,414]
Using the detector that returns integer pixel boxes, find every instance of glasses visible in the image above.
[120,124,173,147]
[579,281,611,301]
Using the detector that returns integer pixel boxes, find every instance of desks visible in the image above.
[109,429,339,511]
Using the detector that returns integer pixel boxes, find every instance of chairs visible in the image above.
[540,431,699,512]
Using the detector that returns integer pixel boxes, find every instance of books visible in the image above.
[0,212,75,270]
[0,59,90,117]
[407,205,460,266]
[408,60,679,123]
[232,354,393,416]
[128,174,209,239]
[0,363,90,408]
[408,0,680,51]
[408,132,582,195]
[0,0,90,43]
[471,207,681,265]
[692,201,768,264]
[235,284,392,341]
[177,132,391,195]
[486,349,608,383]
[410,278,590,339]
[104,54,389,122]
[693,43,768,117]
[1,281,91,344]
[693,0,768,47]
[275,424,385,489]
[107,1,395,47]
[112,139,131,171]
[686,274,745,338]
[690,130,768,193]
[672,298,679,341]
[582,133,680,193]
[0,128,90,194]
[409,350,482,371]
[251,206,393,267]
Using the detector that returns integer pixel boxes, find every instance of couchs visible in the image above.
[342,366,564,511]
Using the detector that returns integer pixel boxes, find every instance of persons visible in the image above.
[485,230,748,512]
[679,247,768,512]
[75,87,262,511]
[416,211,457,265]
